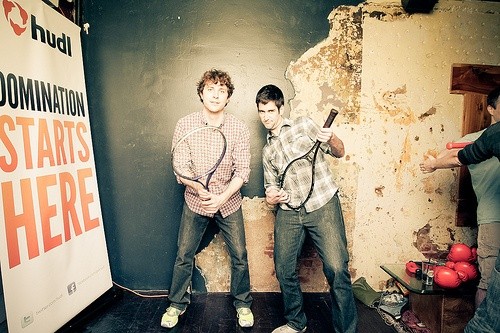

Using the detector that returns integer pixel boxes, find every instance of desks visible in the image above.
[380,264,475,333]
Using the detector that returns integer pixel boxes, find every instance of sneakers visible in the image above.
[160,303,187,329]
[270,322,308,333]
[236,307,255,328]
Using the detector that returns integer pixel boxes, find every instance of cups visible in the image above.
[421,260,436,285]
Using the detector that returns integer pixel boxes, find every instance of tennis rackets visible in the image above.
[280,109,339,210]
[170,125,227,192]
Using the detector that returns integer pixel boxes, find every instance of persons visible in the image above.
[257,84,361,333]
[436,92,500,313]
[161,69,257,328]
[419,119,500,333]
